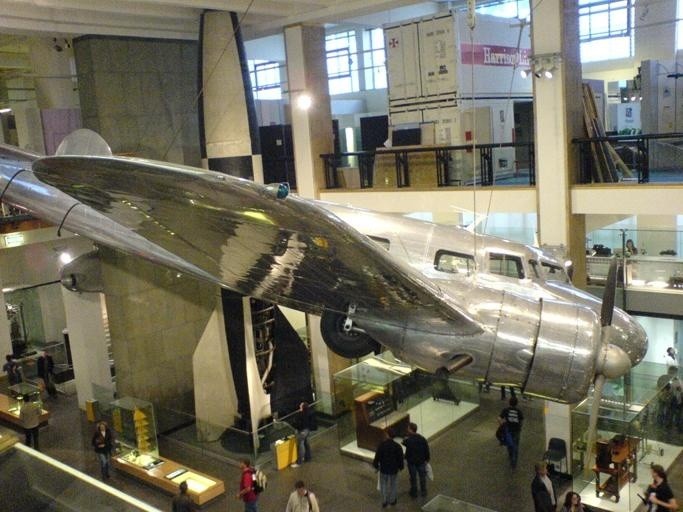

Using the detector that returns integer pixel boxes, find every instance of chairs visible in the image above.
[540,433,570,487]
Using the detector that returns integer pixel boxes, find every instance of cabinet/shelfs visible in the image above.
[594,433,641,505]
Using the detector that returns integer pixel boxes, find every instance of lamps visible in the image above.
[517,50,565,80]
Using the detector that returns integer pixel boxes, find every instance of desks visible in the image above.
[0,392,51,433]
[109,447,227,507]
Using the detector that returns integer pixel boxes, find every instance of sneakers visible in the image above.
[379,498,399,509]
[290,463,302,468]
[419,488,428,499]
[407,487,419,500]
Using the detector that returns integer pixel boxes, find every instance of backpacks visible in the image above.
[251,467,269,495]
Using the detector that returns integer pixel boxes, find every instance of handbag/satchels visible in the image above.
[423,461,435,482]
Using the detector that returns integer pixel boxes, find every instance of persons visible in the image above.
[19,394,42,449]
[91,420,112,481]
[172,481,197,512]
[37,349,58,399]
[2,355,18,385]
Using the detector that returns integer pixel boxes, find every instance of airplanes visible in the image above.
[0,122,658,490]
